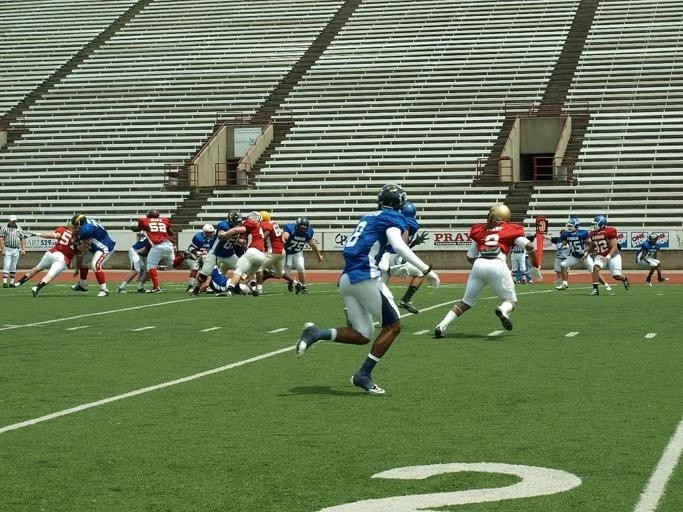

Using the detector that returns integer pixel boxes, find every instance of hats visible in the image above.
[8,214,17,222]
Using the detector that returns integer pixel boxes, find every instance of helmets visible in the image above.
[568,218,580,228]
[64,209,308,232]
[592,215,607,229]
[649,233,658,243]
[377,184,407,210]
[401,202,416,218]
[488,205,511,223]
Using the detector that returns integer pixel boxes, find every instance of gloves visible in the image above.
[426,271,440,289]
[389,264,409,278]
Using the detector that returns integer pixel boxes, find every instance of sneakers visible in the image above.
[3,279,162,297]
[495,305,513,331]
[296,322,318,358]
[351,370,385,395]
[184,250,196,260]
[186,268,308,297]
[435,325,445,338]
[398,300,418,314]
[512,277,670,296]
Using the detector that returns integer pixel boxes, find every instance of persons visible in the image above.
[435,202,542,337]
[0,215,26,288]
[65,209,324,297]
[343,201,430,327]
[13,221,79,298]
[295,184,440,395]
[635,232,669,287]
[511,215,631,295]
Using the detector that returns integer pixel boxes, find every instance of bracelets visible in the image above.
[21,249,25,251]
[419,267,433,275]
